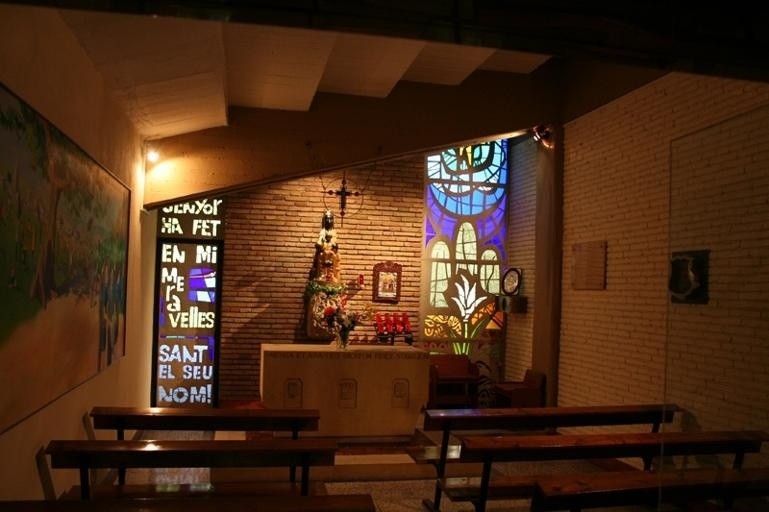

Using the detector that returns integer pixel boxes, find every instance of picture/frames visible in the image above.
[372,261,404,305]
[2,81,134,443]
[497,268,523,295]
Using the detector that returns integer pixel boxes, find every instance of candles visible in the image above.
[373,309,411,335]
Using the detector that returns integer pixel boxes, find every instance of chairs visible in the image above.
[428,350,480,408]
[495,369,547,408]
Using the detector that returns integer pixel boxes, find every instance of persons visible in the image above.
[321,234,336,264]
[312,210,340,284]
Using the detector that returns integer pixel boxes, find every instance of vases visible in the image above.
[335,328,351,349]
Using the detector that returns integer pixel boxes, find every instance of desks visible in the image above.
[259,343,430,446]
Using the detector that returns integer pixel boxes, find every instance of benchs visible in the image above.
[423,397,769,512]
[0,395,375,512]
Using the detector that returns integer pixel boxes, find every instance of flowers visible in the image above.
[313,296,377,345]
